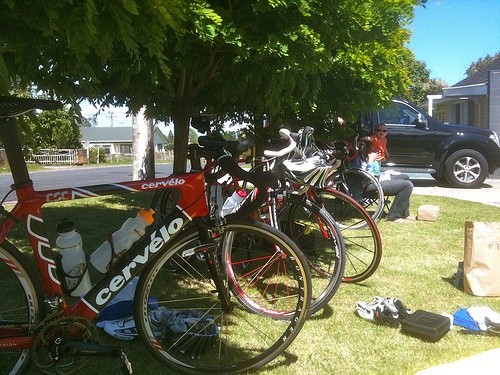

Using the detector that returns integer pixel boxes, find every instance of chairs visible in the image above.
[336,149,391,219]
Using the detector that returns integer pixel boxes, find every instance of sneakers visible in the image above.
[373,296,412,318]
[355,301,398,326]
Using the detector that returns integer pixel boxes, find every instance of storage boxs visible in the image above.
[417,206,440,221]
[400,309,450,342]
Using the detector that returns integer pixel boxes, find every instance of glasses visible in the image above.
[375,129,388,132]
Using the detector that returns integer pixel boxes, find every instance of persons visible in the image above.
[359,125,390,177]
[351,128,416,223]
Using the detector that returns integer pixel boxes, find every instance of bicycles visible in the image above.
[1,92,385,375]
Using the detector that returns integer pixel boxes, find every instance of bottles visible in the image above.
[89,208,156,273]
[57,218,90,297]
[220,189,246,219]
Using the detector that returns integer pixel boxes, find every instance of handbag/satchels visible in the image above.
[463,220,500,297]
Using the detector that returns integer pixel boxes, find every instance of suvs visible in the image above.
[373,97,500,189]
